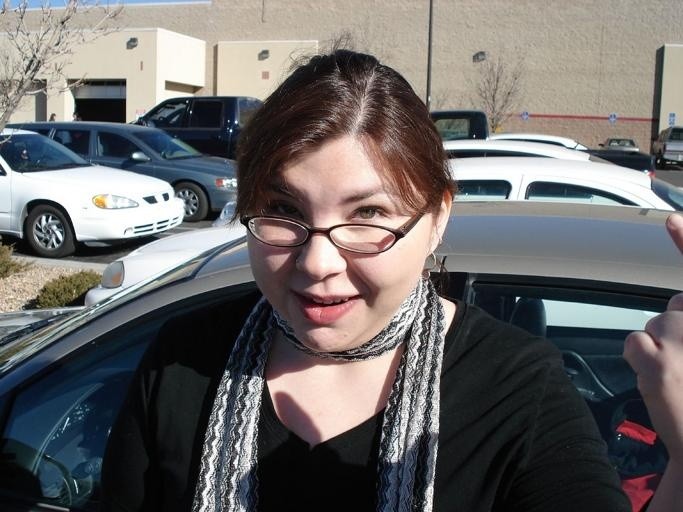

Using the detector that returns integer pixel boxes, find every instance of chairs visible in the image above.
[510,294,551,340]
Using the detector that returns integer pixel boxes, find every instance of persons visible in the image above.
[49,113,56,122]
[90,47,683,512]
[72,111,81,120]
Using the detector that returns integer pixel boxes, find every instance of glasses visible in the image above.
[235,197,439,255]
